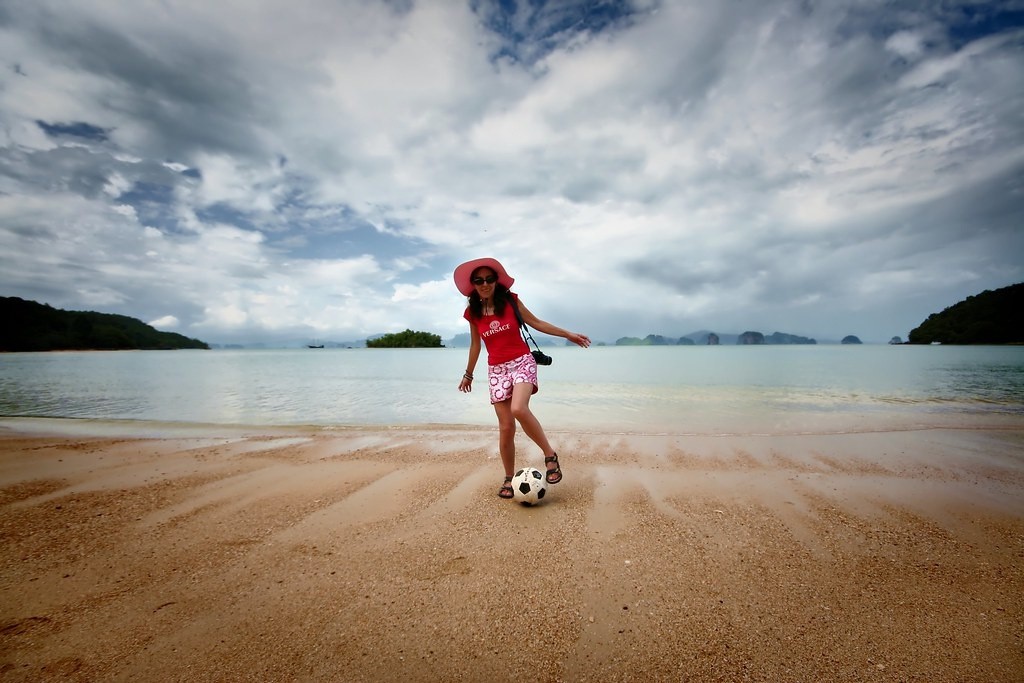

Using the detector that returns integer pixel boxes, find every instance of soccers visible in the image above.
[511,467,548,507]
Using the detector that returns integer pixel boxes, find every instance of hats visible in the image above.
[454,258,515,297]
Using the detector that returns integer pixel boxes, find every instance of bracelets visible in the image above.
[464,370,473,381]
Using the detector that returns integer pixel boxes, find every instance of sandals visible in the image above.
[499,478,514,498]
[545,452,562,484]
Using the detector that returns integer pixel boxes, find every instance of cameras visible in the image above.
[531,351,552,366]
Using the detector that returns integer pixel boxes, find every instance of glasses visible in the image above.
[473,276,496,285]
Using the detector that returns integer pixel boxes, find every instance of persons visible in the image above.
[454,258,591,499]
